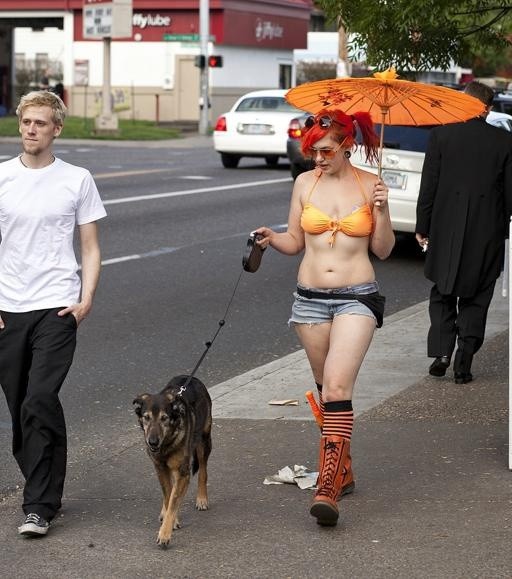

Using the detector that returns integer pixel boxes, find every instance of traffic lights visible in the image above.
[194,54,205,68]
[208,55,222,68]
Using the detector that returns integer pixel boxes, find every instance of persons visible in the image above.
[284,67,486,206]
[0,90,108,535]
[258,108,395,528]
[414,82,512,384]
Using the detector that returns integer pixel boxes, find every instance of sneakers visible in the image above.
[18,512,50,535]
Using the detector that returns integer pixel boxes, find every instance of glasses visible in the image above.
[304,116,335,127]
[304,136,350,159]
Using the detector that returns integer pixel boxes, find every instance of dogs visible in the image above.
[132,374,214,549]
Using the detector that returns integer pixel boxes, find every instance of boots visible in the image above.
[310,435,355,526]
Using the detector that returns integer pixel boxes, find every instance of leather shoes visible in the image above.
[429,355,473,384]
